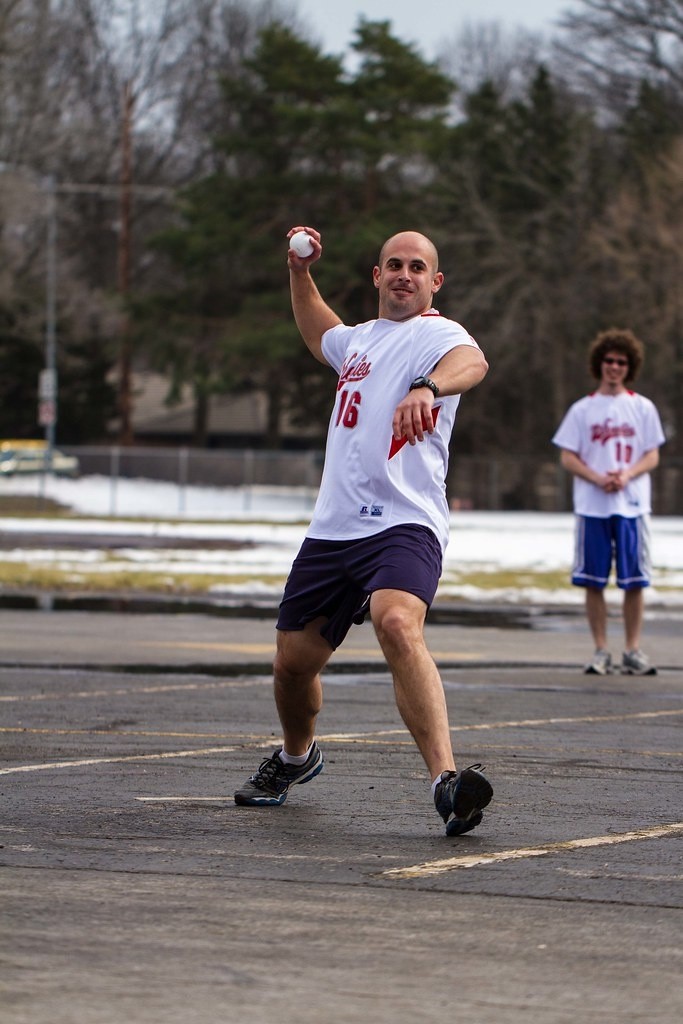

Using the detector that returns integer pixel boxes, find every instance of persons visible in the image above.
[551,330,666,675]
[235,227,494,839]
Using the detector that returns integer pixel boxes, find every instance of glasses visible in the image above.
[603,358,629,366]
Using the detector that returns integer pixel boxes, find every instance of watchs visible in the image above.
[409,376,439,399]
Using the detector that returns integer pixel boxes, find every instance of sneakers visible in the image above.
[585,649,613,674]
[234,740,322,806]
[434,763,493,837]
[621,647,657,674]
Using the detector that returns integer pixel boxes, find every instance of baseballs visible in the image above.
[289,231,315,258]
